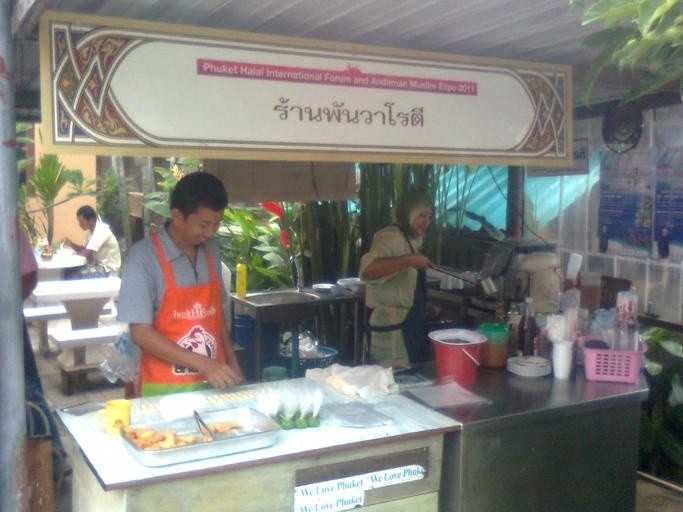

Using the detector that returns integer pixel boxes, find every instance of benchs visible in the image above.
[47,320,130,395]
[22,304,111,357]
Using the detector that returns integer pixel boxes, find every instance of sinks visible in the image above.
[243,291,320,305]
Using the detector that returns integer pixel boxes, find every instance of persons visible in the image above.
[16,222,73,477]
[118,173,246,398]
[360,189,434,372]
[63,206,122,278]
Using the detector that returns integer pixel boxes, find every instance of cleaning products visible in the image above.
[235,261,247,298]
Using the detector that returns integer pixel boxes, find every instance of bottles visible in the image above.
[39,239,52,261]
[476,297,521,366]
[599,224,608,252]
[658,229,669,258]
[516,295,538,353]
[604,301,639,352]
[235,256,247,297]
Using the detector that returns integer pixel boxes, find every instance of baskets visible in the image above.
[578,336,648,383]
[280,346,338,370]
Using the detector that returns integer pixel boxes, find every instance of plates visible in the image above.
[311,277,361,295]
[505,354,553,379]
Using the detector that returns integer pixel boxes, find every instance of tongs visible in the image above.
[191,408,217,441]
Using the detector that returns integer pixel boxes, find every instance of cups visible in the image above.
[545,308,578,342]
[548,338,574,381]
[440,269,498,298]
[564,278,578,293]
[104,398,130,435]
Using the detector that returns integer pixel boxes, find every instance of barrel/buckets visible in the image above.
[427,327,489,388]
[23,400,57,511]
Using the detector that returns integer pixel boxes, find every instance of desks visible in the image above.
[391,361,650,512]
[54,371,462,512]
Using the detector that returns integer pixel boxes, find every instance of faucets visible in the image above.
[296,282,304,293]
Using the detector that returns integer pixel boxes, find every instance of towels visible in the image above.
[306,363,397,401]
